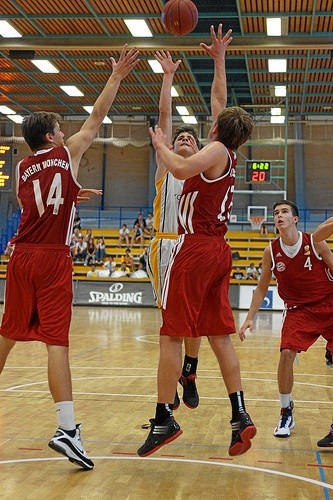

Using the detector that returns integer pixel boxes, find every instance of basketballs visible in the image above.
[162,0,198,36]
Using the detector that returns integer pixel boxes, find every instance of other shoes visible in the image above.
[317,424,333,447]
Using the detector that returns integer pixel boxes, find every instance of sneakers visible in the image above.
[177,373,199,409]
[273,401,296,437]
[228,413,257,457]
[48,423,95,469]
[168,391,180,411]
[137,416,184,457]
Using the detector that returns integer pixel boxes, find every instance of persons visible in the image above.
[135,24,260,456]
[0,44,140,470]
[238,227,333,448]
[5,212,277,282]
[147,48,206,409]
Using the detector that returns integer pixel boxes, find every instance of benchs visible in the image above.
[0,230,333,285]
